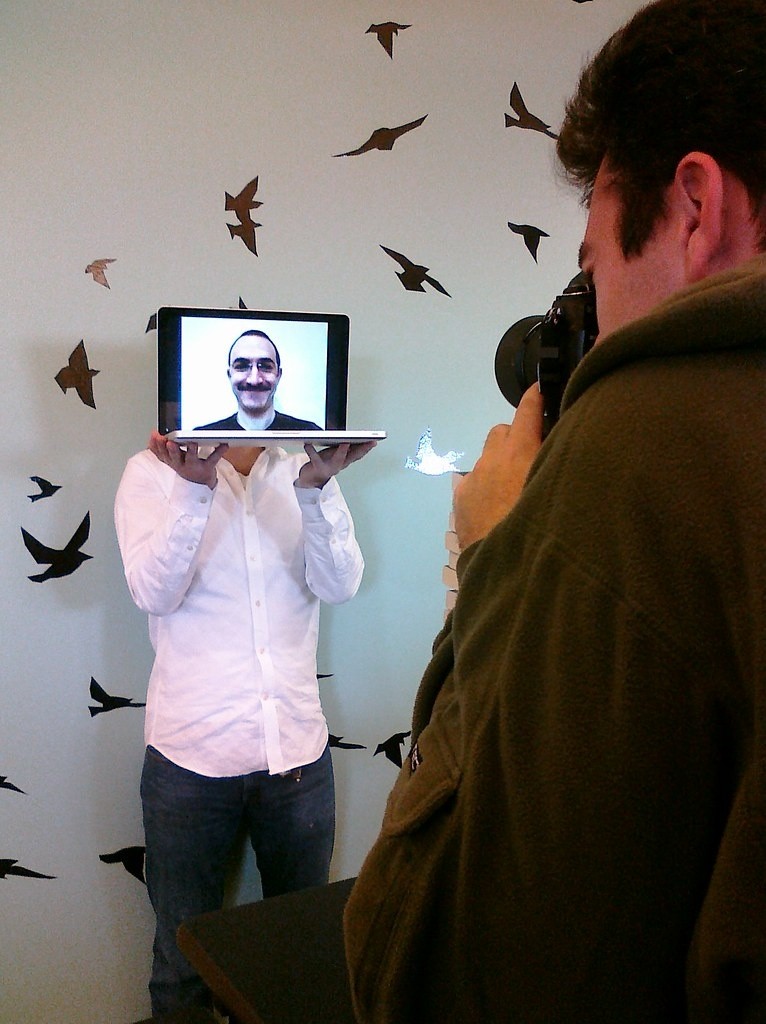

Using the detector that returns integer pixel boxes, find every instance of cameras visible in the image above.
[494,269,601,443]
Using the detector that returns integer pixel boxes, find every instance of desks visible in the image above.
[174,876,359,1024]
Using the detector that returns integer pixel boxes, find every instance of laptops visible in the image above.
[156,308,387,445]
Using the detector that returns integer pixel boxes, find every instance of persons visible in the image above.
[191,330,324,430]
[344,0,766,1024]
[113,429,375,1024]
